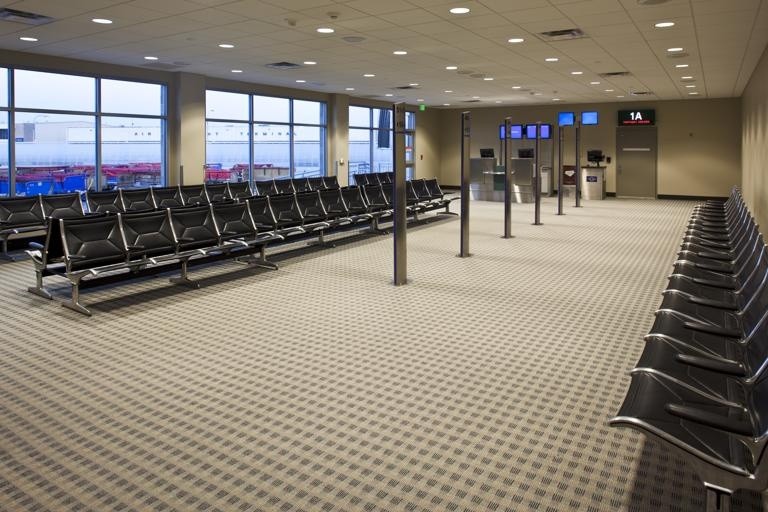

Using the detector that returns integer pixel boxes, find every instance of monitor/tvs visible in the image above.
[518,148,534,158]
[558,112,574,127]
[581,111,598,125]
[526,124,550,139]
[500,125,523,139]
[480,149,494,158]
[587,150,602,161]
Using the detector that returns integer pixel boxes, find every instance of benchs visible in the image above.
[675,185,765,264]
[607,264,766,511]
[0,170,311,317]
[311,171,461,248]
